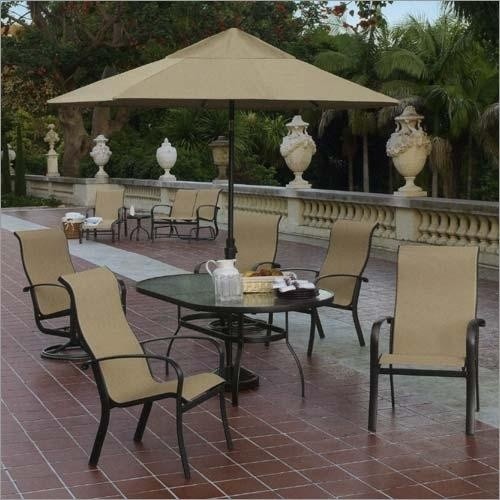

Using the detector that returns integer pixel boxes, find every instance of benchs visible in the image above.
[150,188,222,242]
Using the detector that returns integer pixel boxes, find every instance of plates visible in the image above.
[276,287,320,300]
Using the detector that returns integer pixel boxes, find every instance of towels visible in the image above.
[84,215,103,228]
[65,212,82,221]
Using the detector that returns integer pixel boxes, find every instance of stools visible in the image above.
[61,219,84,240]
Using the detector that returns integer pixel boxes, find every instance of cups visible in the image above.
[214,274,243,300]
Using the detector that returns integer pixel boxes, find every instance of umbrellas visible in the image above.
[47,26,402,394]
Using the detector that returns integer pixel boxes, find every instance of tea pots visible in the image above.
[207,259,239,281]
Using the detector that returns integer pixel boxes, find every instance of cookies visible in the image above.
[249,269,283,277]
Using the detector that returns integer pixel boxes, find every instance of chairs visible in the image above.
[263,217,380,359]
[193,210,281,317]
[13,229,127,360]
[81,187,125,247]
[368,243,487,434]
[57,266,236,483]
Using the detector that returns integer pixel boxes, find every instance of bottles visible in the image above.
[130,206,135,216]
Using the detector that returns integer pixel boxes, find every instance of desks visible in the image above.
[135,268,333,399]
[127,205,151,243]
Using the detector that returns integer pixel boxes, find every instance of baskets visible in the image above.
[243,277,277,293]
